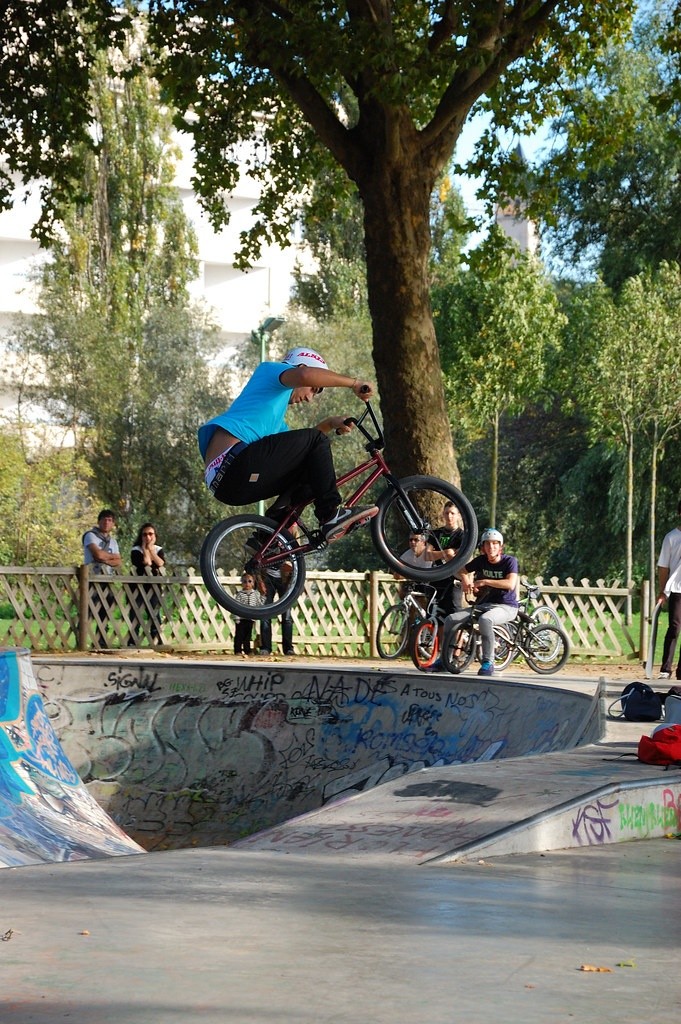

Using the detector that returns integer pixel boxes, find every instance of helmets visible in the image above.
[480,529,504,547]
[281,347,329,395]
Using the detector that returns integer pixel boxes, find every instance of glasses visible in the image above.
[408,538,424,543]
[142,531,155,537]
[242,579,253,583]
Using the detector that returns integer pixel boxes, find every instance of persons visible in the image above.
[125,522,165,647]
[230,574,267,657]
[426,502,475,671]
[393,530,443,658]
[657,500,681,681]
[427,528,519,675]
[255,521,302,654]
[197,346,380,578]
[82,510,121,650]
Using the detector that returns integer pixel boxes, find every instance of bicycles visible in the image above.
[442,578,570,674]
[200,385,479,619]
[376,576,562,671]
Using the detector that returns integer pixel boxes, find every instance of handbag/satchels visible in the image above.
[608,682,664,722]
[638,722,681,768]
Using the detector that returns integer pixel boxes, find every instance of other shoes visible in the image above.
[285,650,295,655]
[235,652,244,656]
[245,651,255,656]
[658,671,670,679]
[259,649,269,655]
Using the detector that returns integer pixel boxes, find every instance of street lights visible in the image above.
[250,317,286,364]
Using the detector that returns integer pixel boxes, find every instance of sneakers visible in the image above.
[246,539,293,571]
[321,501,379,545]
[478,660,494,676]
[425,656,448,672]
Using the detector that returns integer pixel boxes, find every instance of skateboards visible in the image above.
[642,600,662,679]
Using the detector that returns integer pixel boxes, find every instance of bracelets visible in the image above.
[350,377,358,388]
